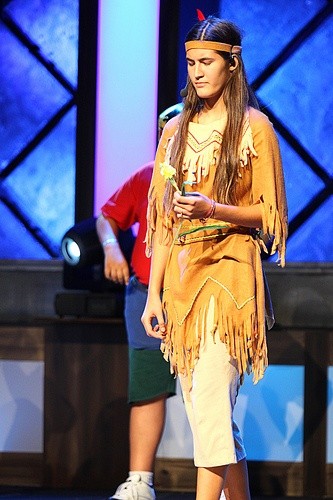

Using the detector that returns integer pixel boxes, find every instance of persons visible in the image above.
[140,14,291,500]
[96,102,227,500]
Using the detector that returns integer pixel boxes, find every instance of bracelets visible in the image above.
[101,238,117,249]
[200,198,216,225]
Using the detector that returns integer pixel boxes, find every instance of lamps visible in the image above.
[54,218,139,319]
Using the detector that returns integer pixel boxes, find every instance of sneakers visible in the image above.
[107,475,155,500]
